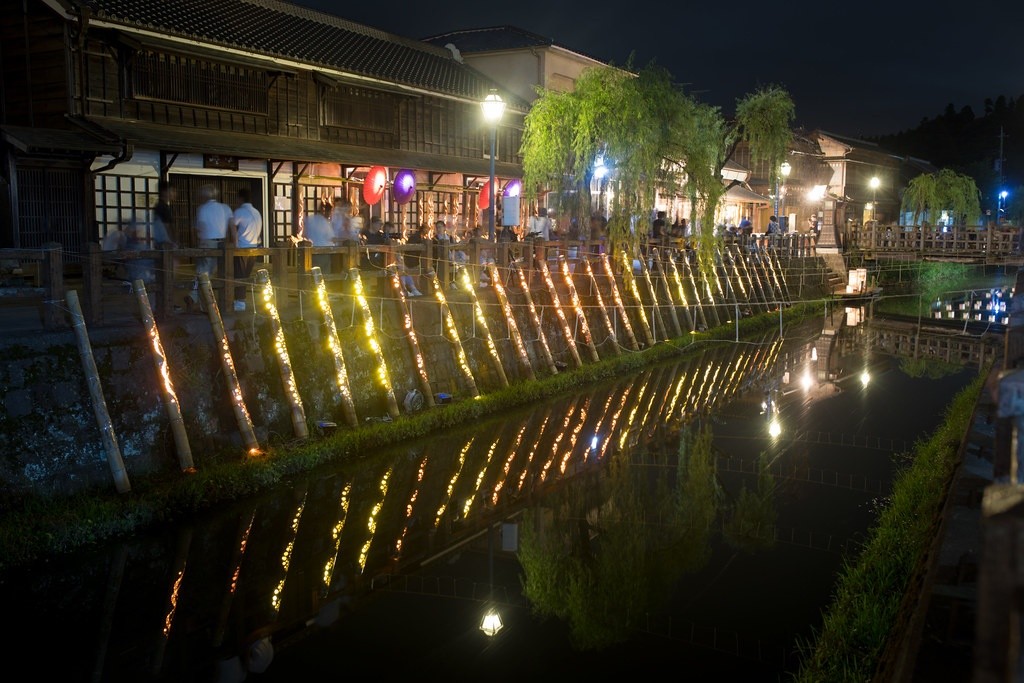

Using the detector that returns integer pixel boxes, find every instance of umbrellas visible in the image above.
[477,177,500,210]
[362,167,388,206]
[501,178,522,206]
[393,169,417,204]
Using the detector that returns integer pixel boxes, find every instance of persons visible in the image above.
[766,215,780,235]
[305,196,753,300]
[184,184,234,316]
[881,214,1024,256]
[807,214,821,246]
[231,189,263,311]
[101,182,176,283]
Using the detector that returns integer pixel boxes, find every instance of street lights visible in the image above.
[478,88,508,258]
[869,177,880,248]
[1001,191,1008,223]
[781,161,792,216]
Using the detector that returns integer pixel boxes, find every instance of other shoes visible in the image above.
[411,289,422,296]
[184,290,201,315]
[479,274,490,280]
[451,283,457,290]
[329,294,341,302]
[479,282,488,288]
[403,290,414,299]
[233,300,246,313]
